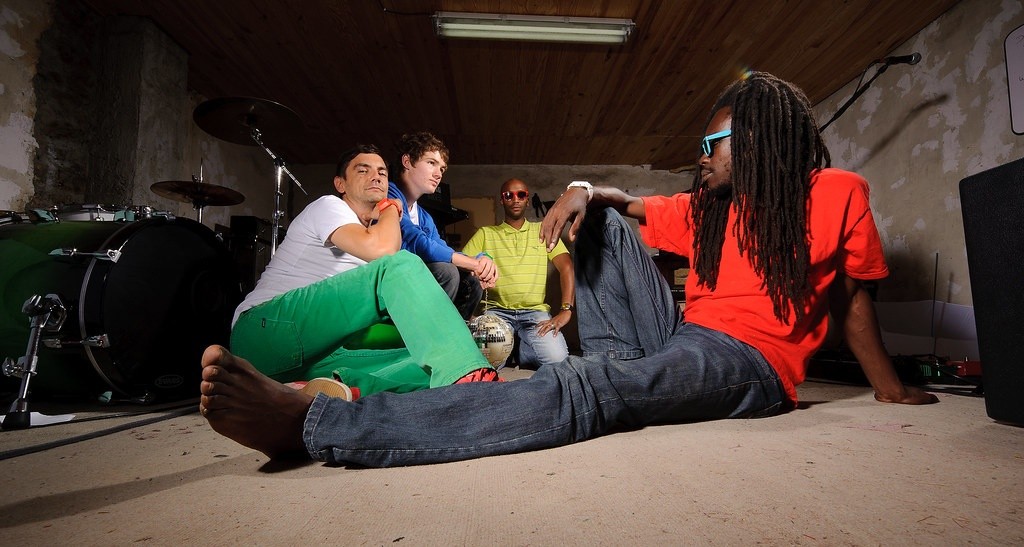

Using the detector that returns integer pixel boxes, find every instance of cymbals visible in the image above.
[149,180,246,206]
[192,95,304,146]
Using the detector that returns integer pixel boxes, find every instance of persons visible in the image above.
[383,131,498,320]
[200,68,938,469]
[461,179,575,369]
[228,142,507,403]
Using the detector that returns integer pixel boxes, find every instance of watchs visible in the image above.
[567,180,594,202]
[378,199,402,217]
[560,303,574,311]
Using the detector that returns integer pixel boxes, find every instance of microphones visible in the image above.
[878,52,922,65]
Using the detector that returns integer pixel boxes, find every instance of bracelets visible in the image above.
[476,253,494,261]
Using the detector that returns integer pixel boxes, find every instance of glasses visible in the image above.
[701,129,732,156]
[501,189,529,202]
[337,144,376,162]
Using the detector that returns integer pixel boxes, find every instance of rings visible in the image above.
[551,324,554,329]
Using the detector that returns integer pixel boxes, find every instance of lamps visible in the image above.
[431,10,637,44]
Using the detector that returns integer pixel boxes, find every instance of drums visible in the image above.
[3,209,250,407]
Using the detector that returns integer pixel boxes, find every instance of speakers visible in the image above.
[958,157,1024,430]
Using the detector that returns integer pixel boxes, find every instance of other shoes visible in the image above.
[283,377,360,401]
[454,368,505,384]
